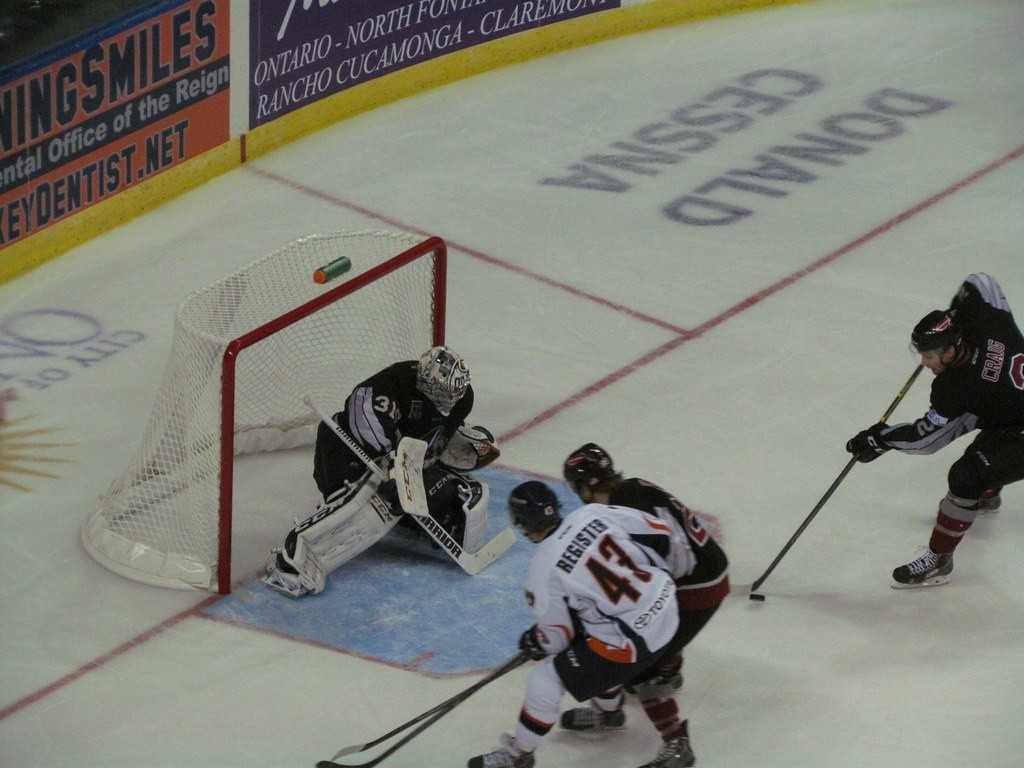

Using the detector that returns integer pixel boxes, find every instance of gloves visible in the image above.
[518,624,548,661]
[846,423,893,463]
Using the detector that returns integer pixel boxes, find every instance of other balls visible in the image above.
[749,594,766,601]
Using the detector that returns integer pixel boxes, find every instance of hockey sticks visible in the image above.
[302,392,519,578]
[329,656,531,763]
[315,650,529,768]
[725,361,927,597]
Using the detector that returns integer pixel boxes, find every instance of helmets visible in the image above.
[564,444,618,496]
[508,481,560,532]
[416,346,471,417]
[910,309,968,369]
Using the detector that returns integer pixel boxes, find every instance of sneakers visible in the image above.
[638,718,695,768]
[624,657,682,699]
[559,698,627,734]
[468,733,535,768]
[977,489,1000,514]
[889,544,955,588]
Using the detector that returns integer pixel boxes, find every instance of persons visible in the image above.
[559,442,731,731]
[468,480,696,768]
[846,273,1024,588]
[262,345,500,599]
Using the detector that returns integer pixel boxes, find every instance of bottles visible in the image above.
[313,256,351,284]
